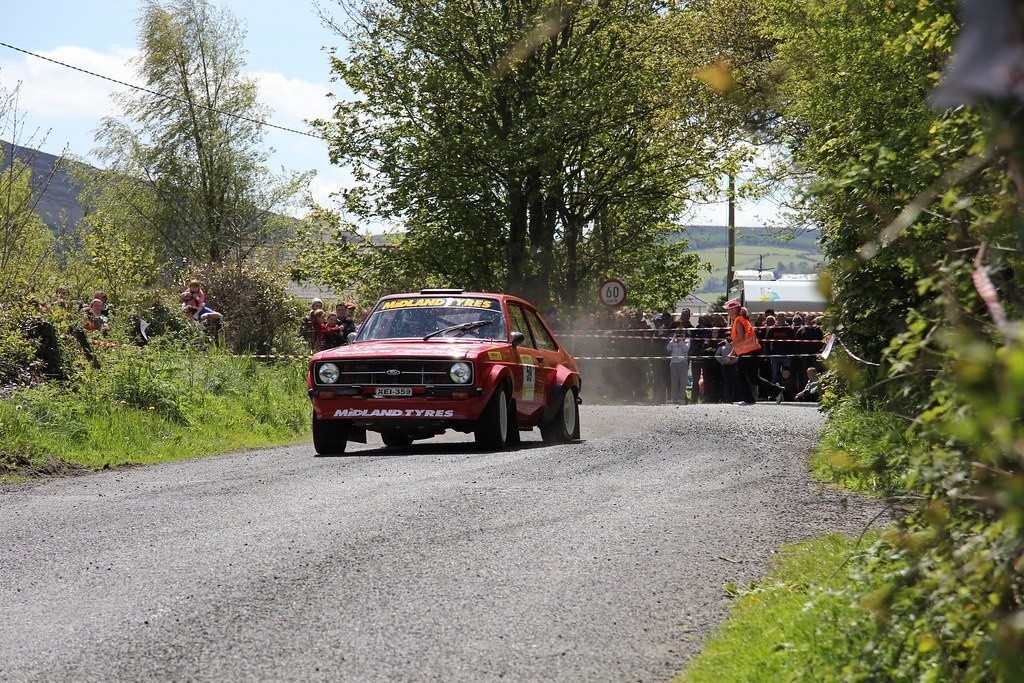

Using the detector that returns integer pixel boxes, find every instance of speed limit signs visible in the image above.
[599,279,627,307]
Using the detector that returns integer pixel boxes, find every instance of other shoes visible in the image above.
[583,387,809,405]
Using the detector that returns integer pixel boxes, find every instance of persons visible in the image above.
[80,291,111,331]
[311,298,368,351]
[51,287,69,309]
[179,279,222,322]
[546,301,831,406]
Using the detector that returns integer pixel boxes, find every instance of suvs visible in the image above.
[307,288,581,455]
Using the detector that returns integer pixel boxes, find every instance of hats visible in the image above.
[312,298,323,305]
[722,301,740,310]
[346,303,356,309]
